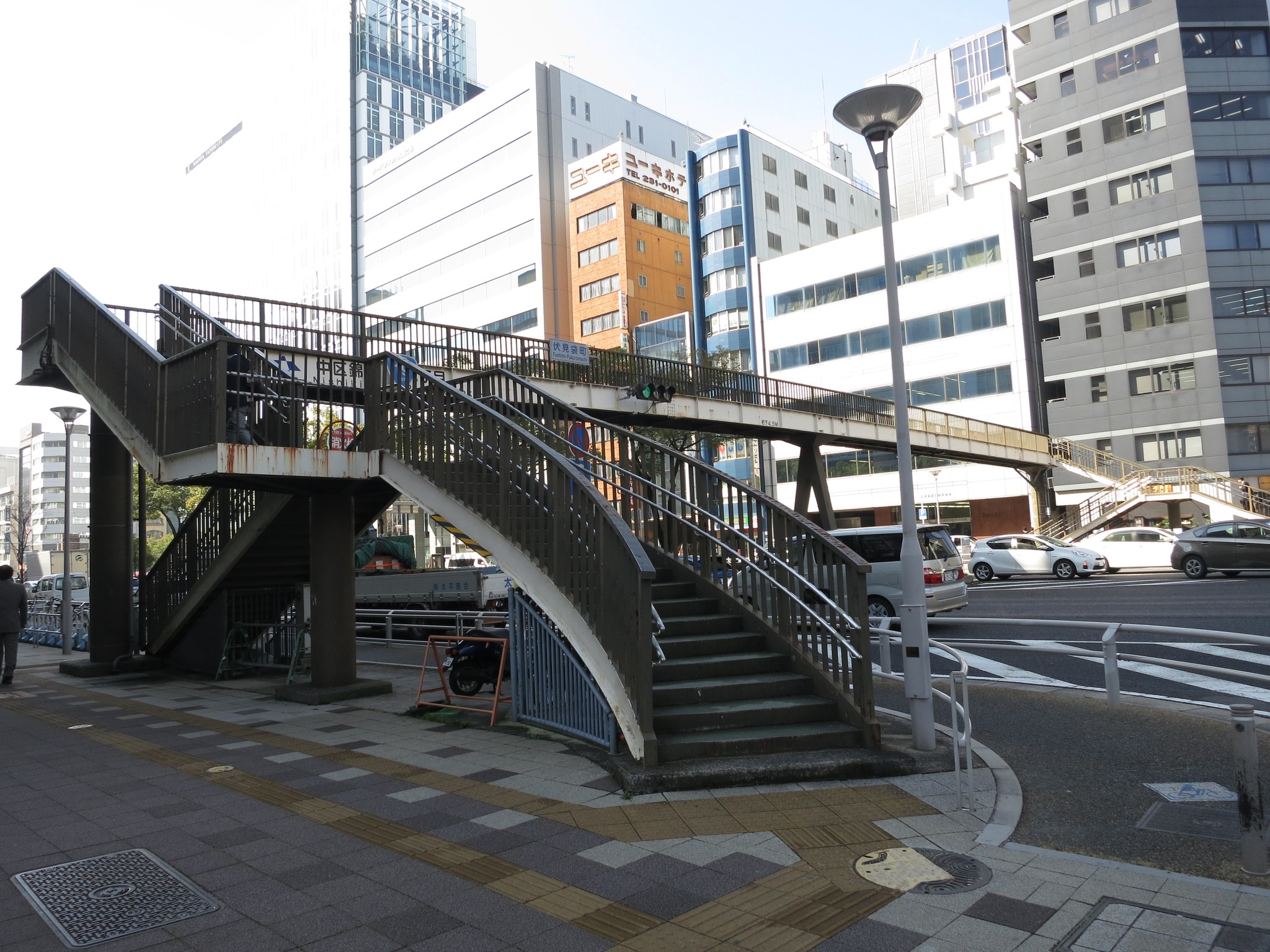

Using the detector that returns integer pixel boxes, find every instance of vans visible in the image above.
[32,572,90,602]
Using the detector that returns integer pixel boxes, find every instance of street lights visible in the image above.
[832,83,946,748]
[50,406,87,655]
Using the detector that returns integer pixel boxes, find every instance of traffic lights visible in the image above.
[634,382,676,403]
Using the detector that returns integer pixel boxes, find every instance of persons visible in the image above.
[0,565,28,685]
[226,341,259,446]
[1160,523,1164,528]
[1019,527,1035,549]
[1237,476,1261,514]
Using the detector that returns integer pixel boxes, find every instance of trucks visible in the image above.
[354,562,519,641]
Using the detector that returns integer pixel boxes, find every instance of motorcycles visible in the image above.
[441,628,513,696]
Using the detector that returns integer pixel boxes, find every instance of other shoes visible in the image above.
[2,676,12,684]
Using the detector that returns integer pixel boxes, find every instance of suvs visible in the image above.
[967,534,1107,581]
[1170,519,1270,579]
[723,524,970,631]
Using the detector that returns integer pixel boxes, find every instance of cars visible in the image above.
[676,555,740,586]
[1071,526,1179,575]
[133,578,140,606]
[22,580,40,601]
